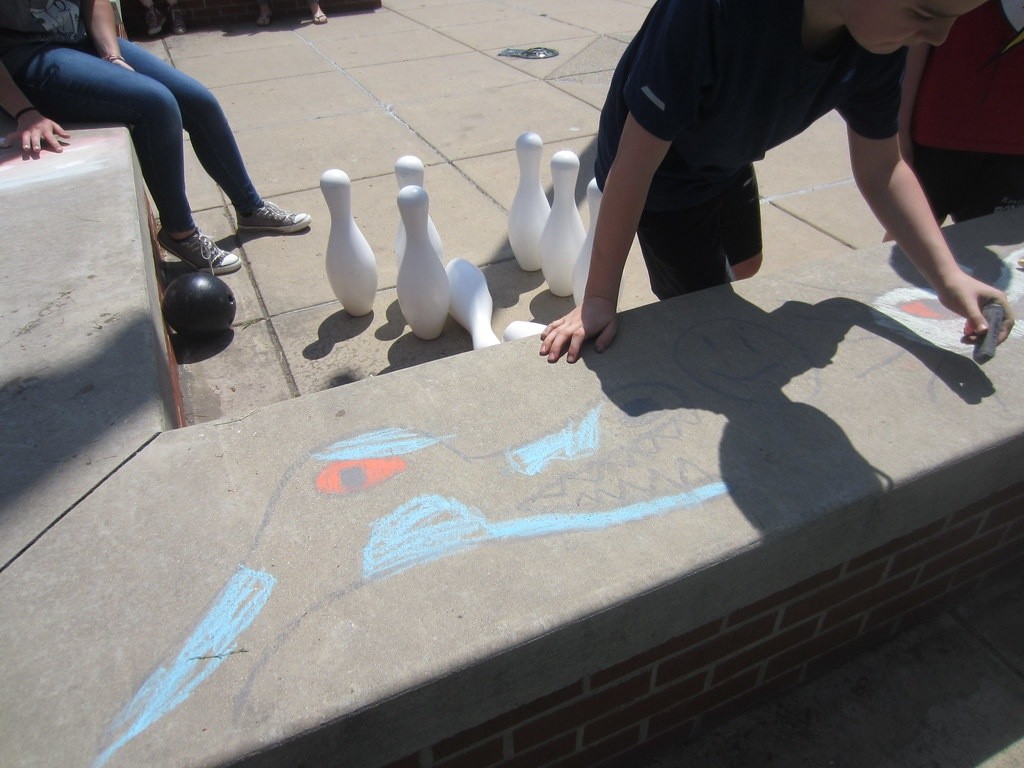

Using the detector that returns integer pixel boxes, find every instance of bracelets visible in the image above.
[14,106,38,121]
[103,55,126,63]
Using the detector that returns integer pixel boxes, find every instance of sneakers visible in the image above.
[143,4,166,35]
[156,227,242,276]
[167,3,188,34]
[237,201,313,233]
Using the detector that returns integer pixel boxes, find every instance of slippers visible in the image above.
[256,15,272,25]
[312,13,327,24]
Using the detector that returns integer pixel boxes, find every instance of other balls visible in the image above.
[162,273,236,337]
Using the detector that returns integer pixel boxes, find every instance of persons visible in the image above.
[882,0,1024,239]
[538,1,1014,363]
[256,0,327,26]
[0,0,310,275]
[140,0,187,34]
